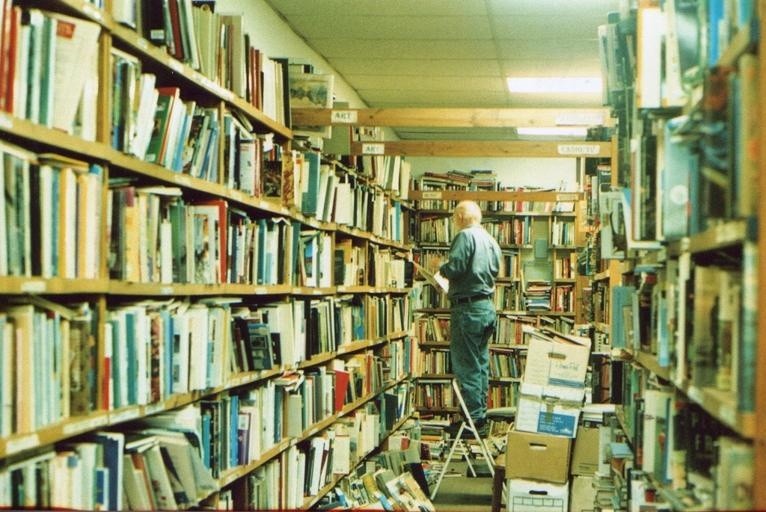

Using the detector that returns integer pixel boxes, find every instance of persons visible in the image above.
[429,200,502,439]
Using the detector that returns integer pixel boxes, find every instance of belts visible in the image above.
[457,294,492,303]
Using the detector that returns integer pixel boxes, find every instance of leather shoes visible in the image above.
[444,417,487,432]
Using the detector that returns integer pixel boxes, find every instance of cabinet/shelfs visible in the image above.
[414,187,578,422]
[576,1,766,511]
[3,0,417,510]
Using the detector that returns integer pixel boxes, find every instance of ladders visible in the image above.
[430,378,507,504]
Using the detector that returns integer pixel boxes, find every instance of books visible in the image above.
[443,439,492,461]
[479,0,766,512]
[0,1,460,511]
[447,169,496,192]
